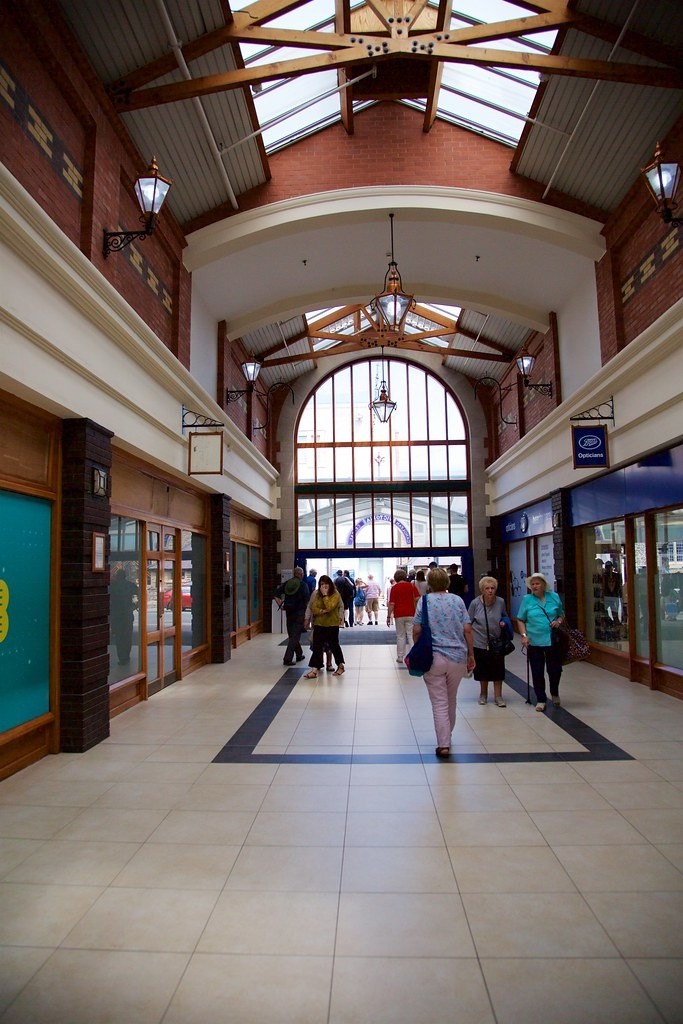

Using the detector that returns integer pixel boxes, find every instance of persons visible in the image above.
[386,562,469,663]
[468,577,508,707]
[274,566,382,678]
[596,557,674,624]
[517,573,565,712]
[412,569,475,758]
[109,570,140,664]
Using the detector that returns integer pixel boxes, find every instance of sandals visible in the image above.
[436,746,449,757]
[326,665,335,672]
[303,671,317,678]
[333,666,345,676]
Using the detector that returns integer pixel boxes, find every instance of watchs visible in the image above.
[558,620,562,625]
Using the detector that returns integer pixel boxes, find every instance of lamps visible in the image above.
[641,141,683,240]
[101,155,176,259]
[369,345,398,423]
[370,213,418,332]
[226,348,263,406]
[513,344,553,401]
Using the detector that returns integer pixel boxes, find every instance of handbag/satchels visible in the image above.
[358,596,366,602]
[550,627,568,654]
[489,635,516,656]
[560,627,591,666]
[403,594,433,677]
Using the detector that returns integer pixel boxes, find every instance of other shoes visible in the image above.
[536,702,546,711]
[397,656,404,663]
[375,621,378,625]
[367,621,373,625]
[495,697,506,707]
[478,694,487,704]
[283,659,297,666]
[296,655,305,662]
[551,695,560,707]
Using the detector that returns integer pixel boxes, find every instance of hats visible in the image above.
[285,577,301,595]
[358,578,362,581]
[337,570,343,575]
[525,572,551,592]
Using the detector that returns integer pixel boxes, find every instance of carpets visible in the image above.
[278,630,410,646]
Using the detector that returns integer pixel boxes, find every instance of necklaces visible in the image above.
[541,599,544,602]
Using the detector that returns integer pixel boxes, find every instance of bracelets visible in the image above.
[521,634,527,638]
[387,617,390,618]
